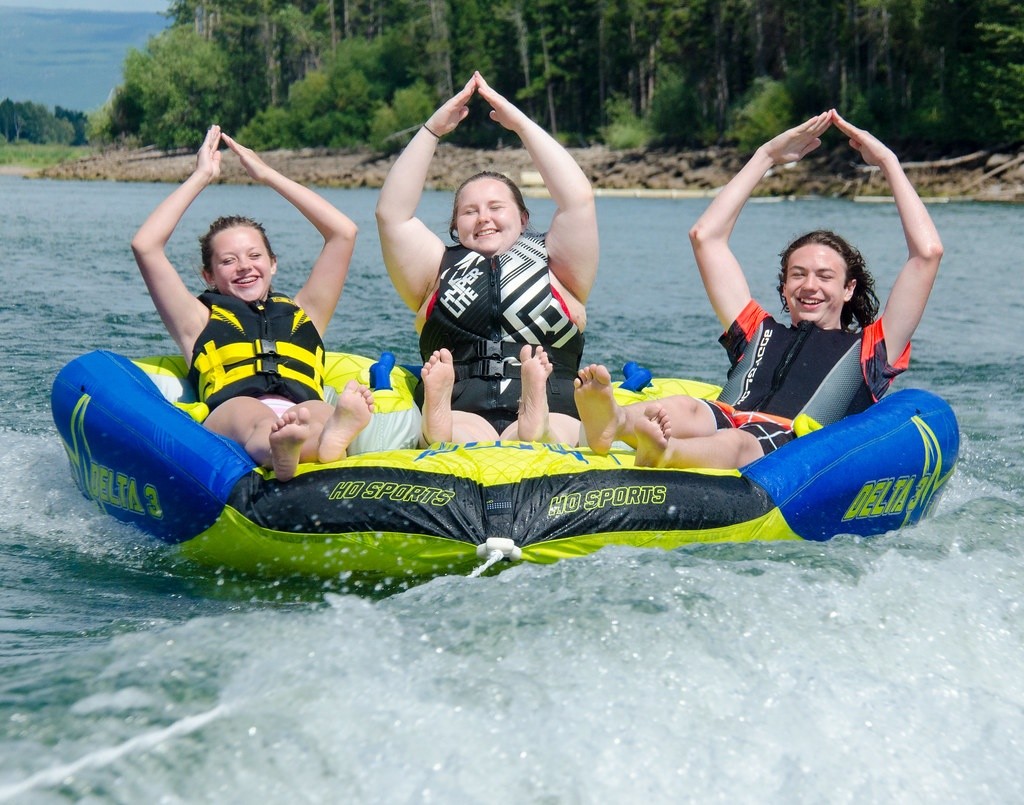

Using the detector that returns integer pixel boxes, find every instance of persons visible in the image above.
[572,107,945,469]
[130,122,376,481]
[374,70,600,450]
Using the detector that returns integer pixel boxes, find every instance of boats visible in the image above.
[47,343,968,579]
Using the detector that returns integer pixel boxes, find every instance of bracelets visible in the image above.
[423,124,440,138]
